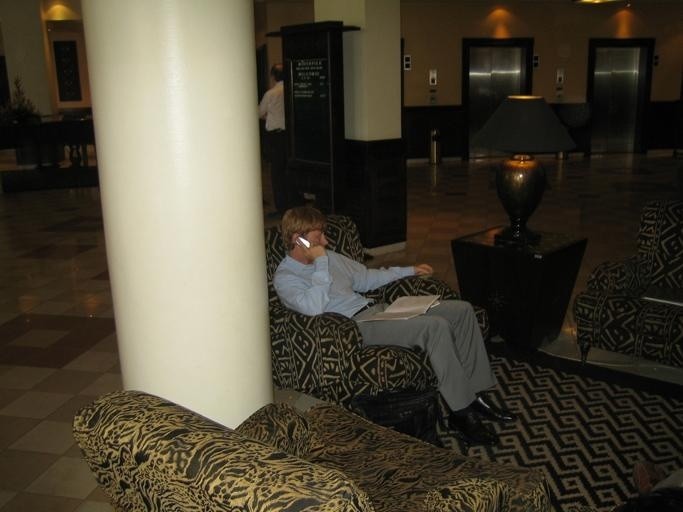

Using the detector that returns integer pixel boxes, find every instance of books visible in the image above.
[356,294,439,322]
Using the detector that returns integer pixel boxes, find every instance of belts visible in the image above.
[346,299,380,318]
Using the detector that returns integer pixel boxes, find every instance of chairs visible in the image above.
[264,215,489,448]
[573,200,683,370]
[73,389,552,512]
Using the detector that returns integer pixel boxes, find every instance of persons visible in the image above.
[257,63,286,216]
[273,207,513,445]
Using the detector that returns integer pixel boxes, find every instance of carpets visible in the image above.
[391,338,683,512]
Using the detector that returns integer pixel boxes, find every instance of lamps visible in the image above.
[470,95,577,247]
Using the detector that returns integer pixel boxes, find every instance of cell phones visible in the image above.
[296,237,310,248]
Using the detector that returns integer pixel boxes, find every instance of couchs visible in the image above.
[549,103,593,160]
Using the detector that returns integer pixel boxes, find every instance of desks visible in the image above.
[0,108,95,169]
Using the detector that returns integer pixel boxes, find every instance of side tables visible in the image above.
[451,225,589,348]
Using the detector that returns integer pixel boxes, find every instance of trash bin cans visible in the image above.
[426,128,441,165]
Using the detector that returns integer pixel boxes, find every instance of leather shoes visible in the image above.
[467,394,515,422]
[448,411,500,446]
